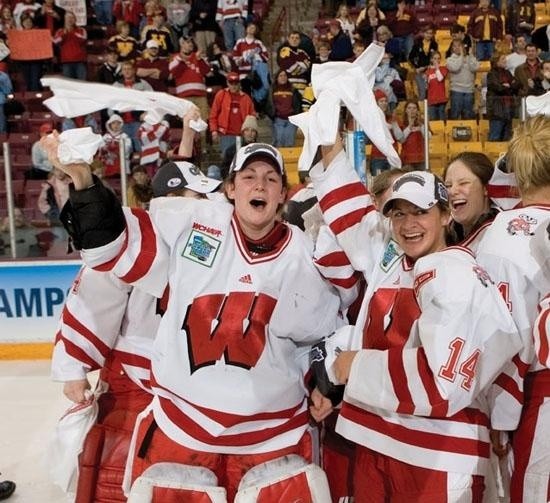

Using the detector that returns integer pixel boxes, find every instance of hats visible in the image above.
[146,39,159,48]
[240,116,258,134]
[226,71,240,81]
[383,170,450,216]
[151,160,223,196]
[228,141,285,175]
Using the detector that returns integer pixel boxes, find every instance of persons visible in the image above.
[36,105,549,503]
[1,0,550,234]
